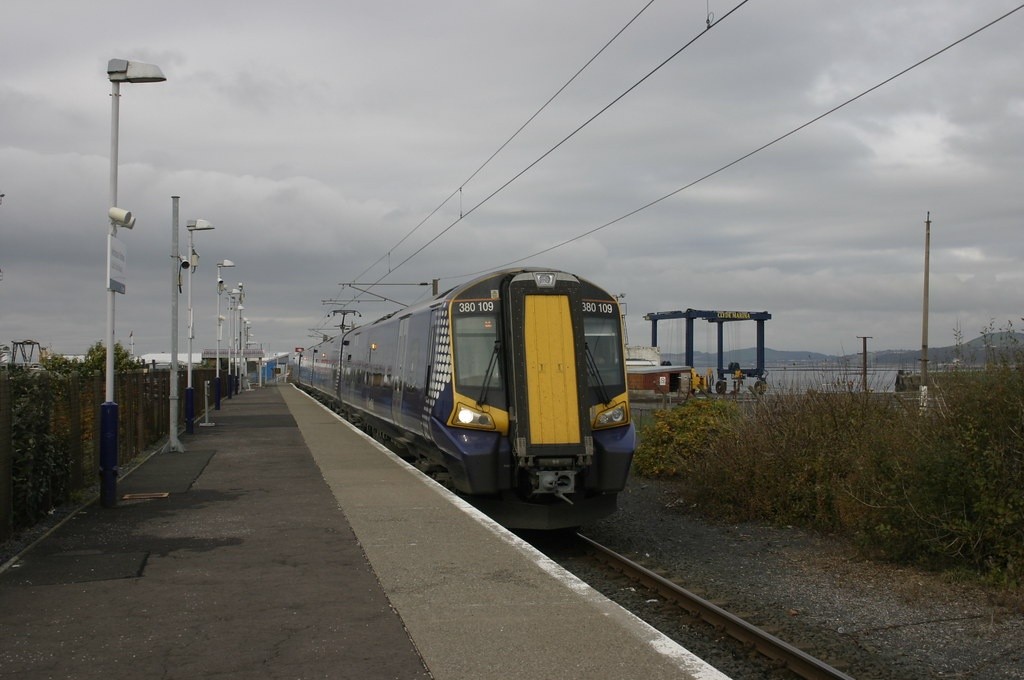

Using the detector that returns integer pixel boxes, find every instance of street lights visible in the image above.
[185,218,216,434]
[226,288,242,400]
[235,305,254,396]
[99,58,168,511]
[215,258,236,411]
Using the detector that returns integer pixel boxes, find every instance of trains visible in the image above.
[288,264,637,529]
